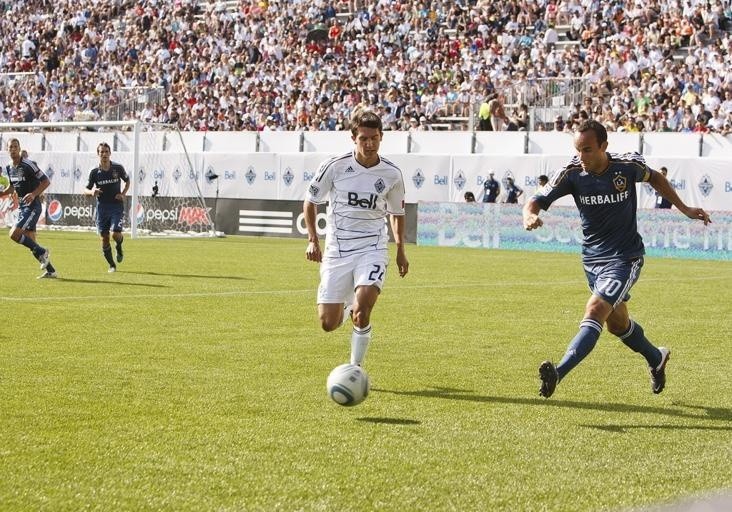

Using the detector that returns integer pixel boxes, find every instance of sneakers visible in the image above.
[35,269,58,279]
[105,252,125,277]
[34,244,54,269]
[649,343,674,396]
[536,360,558,401]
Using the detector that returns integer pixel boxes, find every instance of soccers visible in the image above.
[327,364,368,406]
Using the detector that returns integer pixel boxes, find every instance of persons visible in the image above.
[523,121,714,399]
[0,139,57,279]
[85,143,130,272]
[304,113,409,367]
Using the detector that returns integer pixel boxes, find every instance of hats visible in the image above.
[409,115,427,123]
